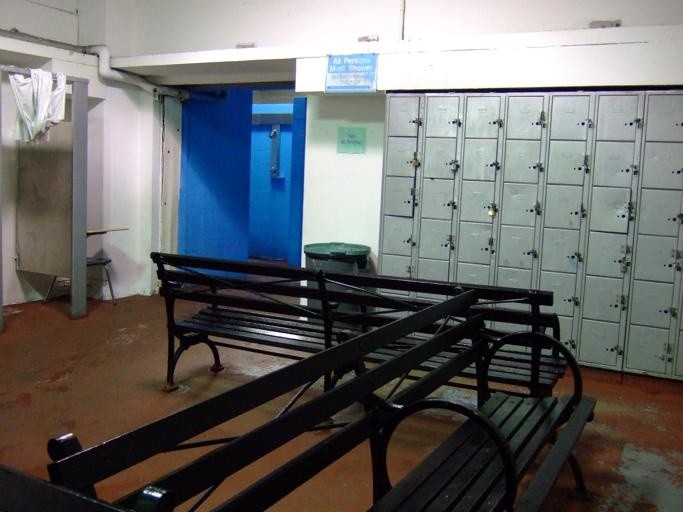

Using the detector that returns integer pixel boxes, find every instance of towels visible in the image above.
[304,242,371,333]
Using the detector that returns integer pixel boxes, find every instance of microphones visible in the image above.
[42,258,116,306]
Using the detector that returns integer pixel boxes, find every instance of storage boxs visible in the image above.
[0,464,176,512]
[47,289,598,512]
[151,252,568,444]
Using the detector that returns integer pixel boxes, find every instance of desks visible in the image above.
[87,227,129,236]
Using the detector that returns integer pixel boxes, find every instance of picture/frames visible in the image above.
[87,227,129,236]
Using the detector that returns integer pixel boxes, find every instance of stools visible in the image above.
[42,258,116,306]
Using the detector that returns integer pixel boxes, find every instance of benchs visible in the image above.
[0,464,176,512]
[47,289,598,512]
[151,252,568,444]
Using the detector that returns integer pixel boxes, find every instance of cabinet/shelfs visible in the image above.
[373,90,683,385]
